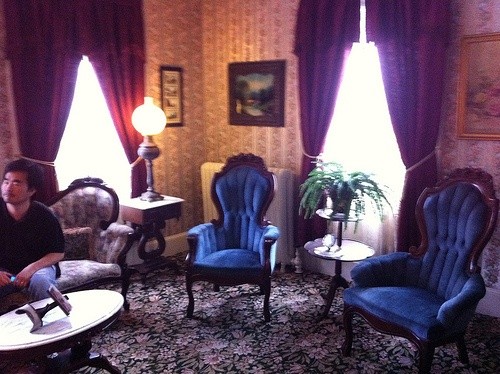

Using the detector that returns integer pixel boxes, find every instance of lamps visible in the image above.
[131,96,166,202]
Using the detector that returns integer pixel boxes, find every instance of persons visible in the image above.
[0,158,65,301]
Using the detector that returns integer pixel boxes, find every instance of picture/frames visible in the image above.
[159,66,183,127]
[226,59,286,127]
[456,32,500,141]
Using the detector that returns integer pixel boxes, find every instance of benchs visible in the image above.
[0,177,134,311]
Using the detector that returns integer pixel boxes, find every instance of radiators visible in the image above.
[200,162,294,265]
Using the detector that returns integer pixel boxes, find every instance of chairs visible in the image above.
[342,167,499,374]
[183,152,280,323]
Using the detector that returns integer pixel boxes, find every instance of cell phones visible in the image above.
[11,276,15,282]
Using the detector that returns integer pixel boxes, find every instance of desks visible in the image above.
[317,208,364,247]
[304,238,376,322]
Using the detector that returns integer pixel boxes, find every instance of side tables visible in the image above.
[119,194,185,290]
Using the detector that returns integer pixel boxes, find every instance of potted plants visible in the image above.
[297,153,393,224]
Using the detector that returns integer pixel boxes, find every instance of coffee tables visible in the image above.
[0,289,125,374]
